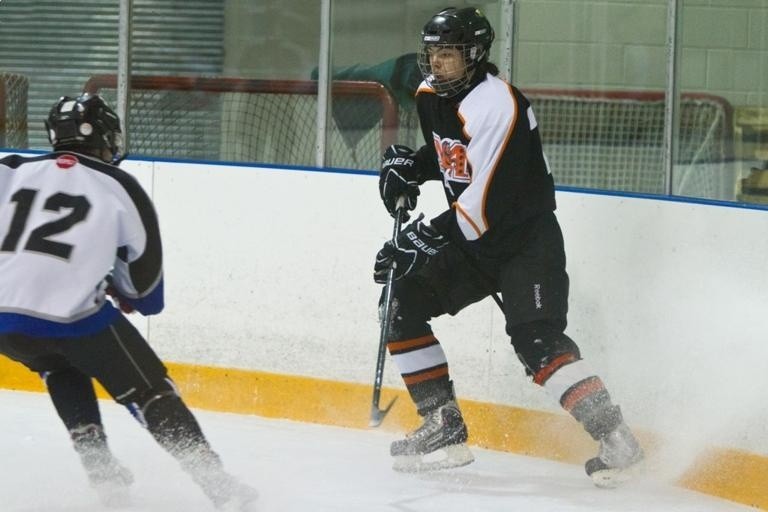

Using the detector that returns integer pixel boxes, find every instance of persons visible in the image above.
[0,95,244,508]
[378,7,645,486]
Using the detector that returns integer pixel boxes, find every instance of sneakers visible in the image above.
[83,451,135,495]
[390,380,468,458]
[584,404,650,481]
[195,467,259,508]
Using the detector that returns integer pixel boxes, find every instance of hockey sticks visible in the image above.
[369,194,398,428]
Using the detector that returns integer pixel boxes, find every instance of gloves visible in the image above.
[379,144,421,224]
[373,211,453,285]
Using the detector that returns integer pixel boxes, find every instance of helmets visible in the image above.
[43,93,129,167]
[418,6,496,99]
